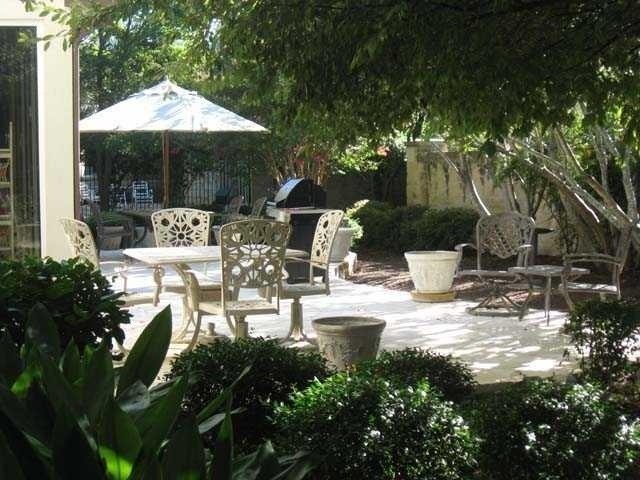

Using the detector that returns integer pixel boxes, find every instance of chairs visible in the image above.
[454,211,640,325]
[227,196,268,216]
[85,197,136,249]
[132,181,153,206]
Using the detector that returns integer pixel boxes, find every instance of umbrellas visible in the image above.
[78,74,273,208]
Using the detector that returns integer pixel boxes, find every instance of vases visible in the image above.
[403,250,459,294]
[311,317,387,368]
